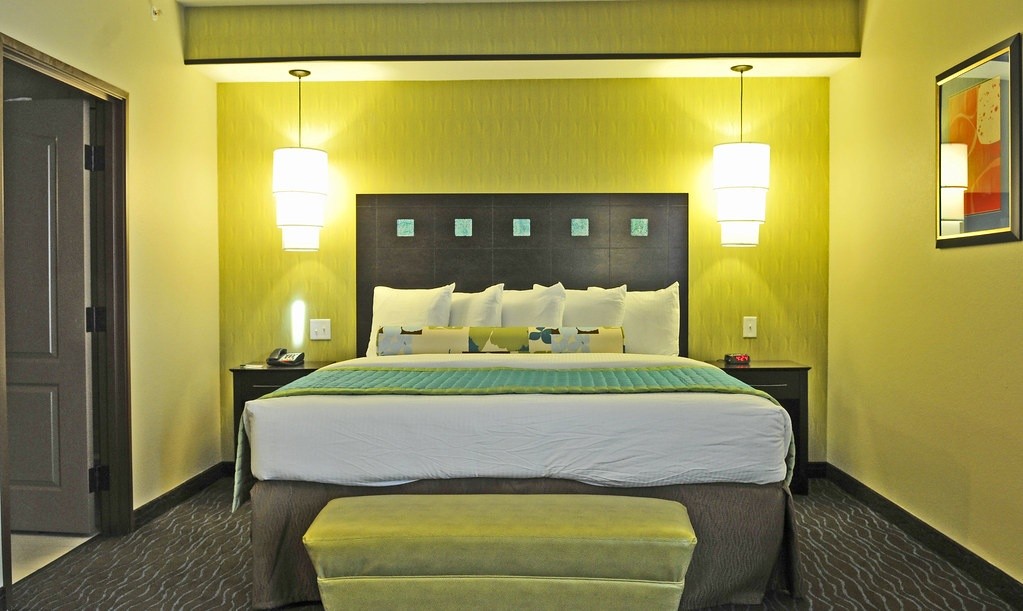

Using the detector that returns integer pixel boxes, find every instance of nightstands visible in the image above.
[705,359,812,496]
[229,360,337,464]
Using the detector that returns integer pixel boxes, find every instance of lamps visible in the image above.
[273,70,329,252]
[712,63,771,249]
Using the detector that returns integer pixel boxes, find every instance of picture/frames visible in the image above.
[936,31,1022,250]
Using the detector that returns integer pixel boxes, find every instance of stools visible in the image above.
[303,493,697,610]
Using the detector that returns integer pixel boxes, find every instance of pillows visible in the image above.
[365,282,457,357]
[501,282,567,327]
[449,283,505,326]
[375,326,627,356]
[588,281,681,357]
[532,284,628,327]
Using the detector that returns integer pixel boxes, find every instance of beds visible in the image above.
[231,357,810,607]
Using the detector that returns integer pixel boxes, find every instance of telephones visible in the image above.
[266,348,304,367]
